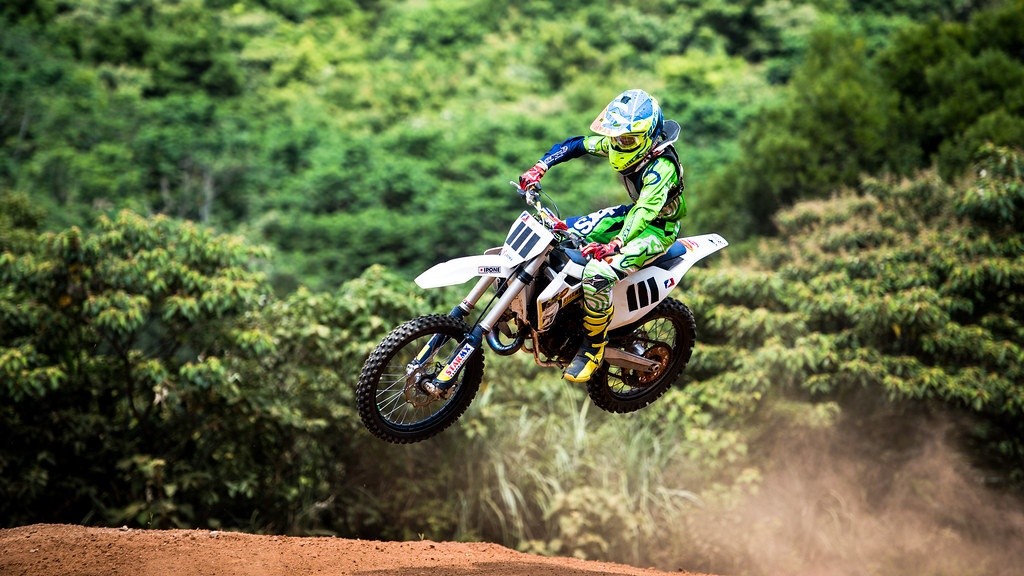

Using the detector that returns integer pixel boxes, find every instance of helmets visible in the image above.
[590,89,663,172]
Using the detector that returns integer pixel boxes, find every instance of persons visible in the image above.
[519,88,687,381]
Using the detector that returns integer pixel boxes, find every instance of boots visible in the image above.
[564,301,614,383]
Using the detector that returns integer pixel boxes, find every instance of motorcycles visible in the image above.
[351,173,731,448]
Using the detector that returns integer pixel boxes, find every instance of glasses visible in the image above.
[612,96,659,151]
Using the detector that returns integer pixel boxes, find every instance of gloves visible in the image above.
[520,163,545,191]
[581,241,621,262]
